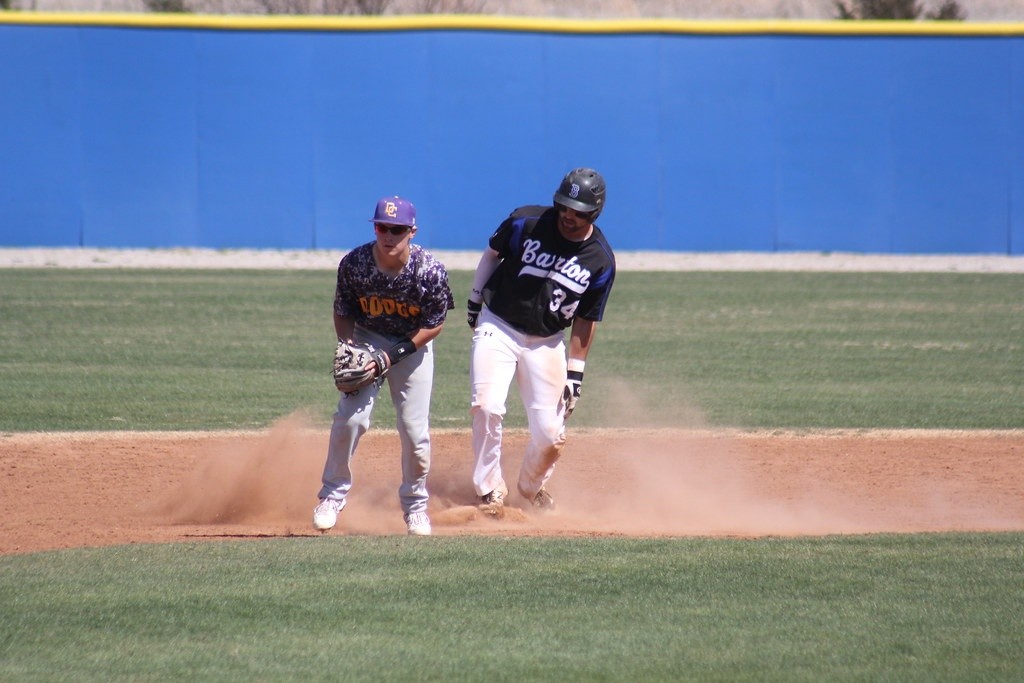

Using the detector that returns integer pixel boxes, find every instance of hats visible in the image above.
[368,196,416,227]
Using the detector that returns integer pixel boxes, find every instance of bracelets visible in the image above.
[386,338,417,366]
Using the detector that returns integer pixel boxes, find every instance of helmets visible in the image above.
[553,168,606,224]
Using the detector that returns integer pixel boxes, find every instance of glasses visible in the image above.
[557,203,588,219]
[374,223,411,235]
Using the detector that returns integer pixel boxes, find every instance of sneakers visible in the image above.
[518,483,555,512]
[403,512,431,536]
[479,478,509,519]
[312,497,347,529]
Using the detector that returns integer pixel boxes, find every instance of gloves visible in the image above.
[467,300,481,331]
[561,358,585,420]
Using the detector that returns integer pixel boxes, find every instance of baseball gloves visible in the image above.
[333,343,390,394]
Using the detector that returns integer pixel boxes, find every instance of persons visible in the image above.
[468,169,616,518]
[312,196,455,535]
